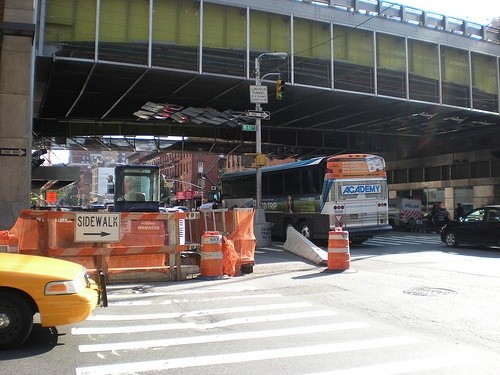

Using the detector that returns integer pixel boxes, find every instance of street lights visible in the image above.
[254,51,287,252]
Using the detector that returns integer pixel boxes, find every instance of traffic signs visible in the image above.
[247,110,270,120]
[74,212,120,245]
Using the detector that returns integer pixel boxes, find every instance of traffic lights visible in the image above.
[213,192,220,202]
[276,79,285,102]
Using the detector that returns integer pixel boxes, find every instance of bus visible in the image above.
[221,153,393,243]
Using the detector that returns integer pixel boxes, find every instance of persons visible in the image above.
[432,203,447,234]
[106,175,115,194]
[57,186,78,207]
[478,211,484,221]
[456,203,464,218]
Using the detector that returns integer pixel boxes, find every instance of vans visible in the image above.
[199,202,219,209]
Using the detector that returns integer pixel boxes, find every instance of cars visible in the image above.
[0,252,100,350]
[440,205,500,249]
[159,205,190,214]
